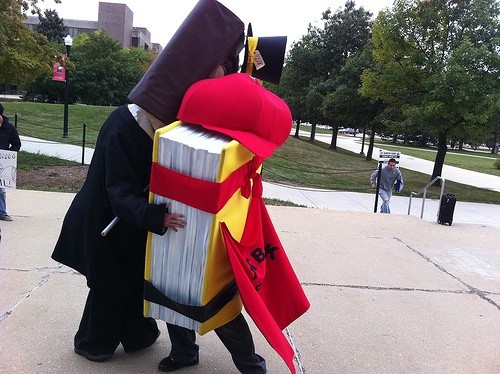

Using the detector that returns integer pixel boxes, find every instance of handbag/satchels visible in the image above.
[393,178,403,193]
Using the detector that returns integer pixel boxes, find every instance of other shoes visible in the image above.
[1,214,13,221]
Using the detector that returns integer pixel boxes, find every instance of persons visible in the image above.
[0,102,22,221]
[50,0,247,362]
[370,158,404,214]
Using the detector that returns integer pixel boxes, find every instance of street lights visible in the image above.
[62,33,73,139]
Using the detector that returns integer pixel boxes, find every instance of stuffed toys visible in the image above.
[143,72,310,374]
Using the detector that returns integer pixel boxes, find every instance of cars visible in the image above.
[365,130,494,151]
[291,120,359,135]
